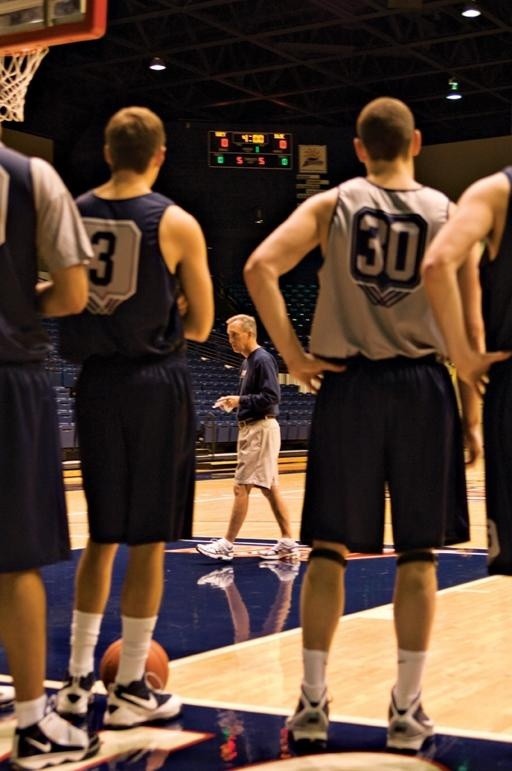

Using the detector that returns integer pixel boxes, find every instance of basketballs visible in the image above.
[100,637,170,694]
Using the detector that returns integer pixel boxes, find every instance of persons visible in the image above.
[195,314,300,563]
[46,106,217,732]
[197,559,301,771]
[419,165,511,580]
[0,141,99,771]
[242,93,485,755]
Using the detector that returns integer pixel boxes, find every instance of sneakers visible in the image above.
[103,670,184,728]
[56,671,97,718]
[285,684,332,750]
[386,684,434,751]
[0,683,15,713]
[195,538,234,561]
[259,539,300,560]
[10,711,102,770]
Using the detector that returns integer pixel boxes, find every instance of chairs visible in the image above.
[39,317,317,449]
